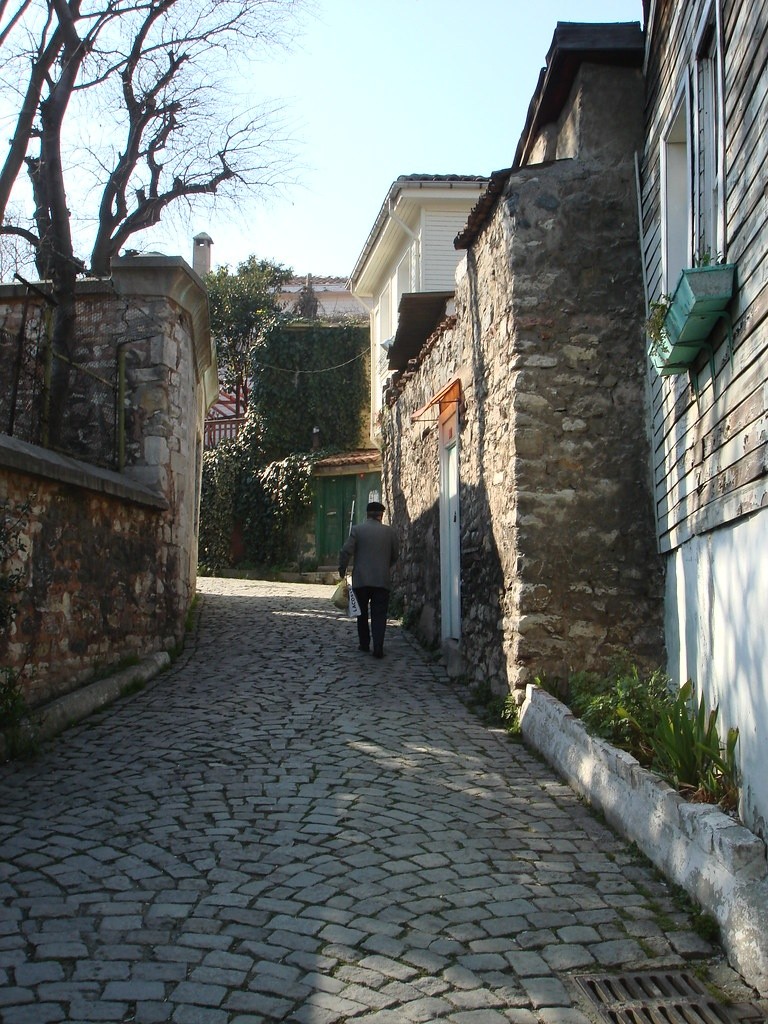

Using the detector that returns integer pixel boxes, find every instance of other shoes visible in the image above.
[358,645,370,651]
[373,648,382,659]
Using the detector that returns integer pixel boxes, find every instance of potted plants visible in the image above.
[642,244,736,377]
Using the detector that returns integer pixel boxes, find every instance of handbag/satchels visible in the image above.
[331,577,349,609]
[346,587,361,618]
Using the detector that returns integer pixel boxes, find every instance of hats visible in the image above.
[367,502,385,511]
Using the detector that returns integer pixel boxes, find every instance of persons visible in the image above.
[338,502,400,659]
